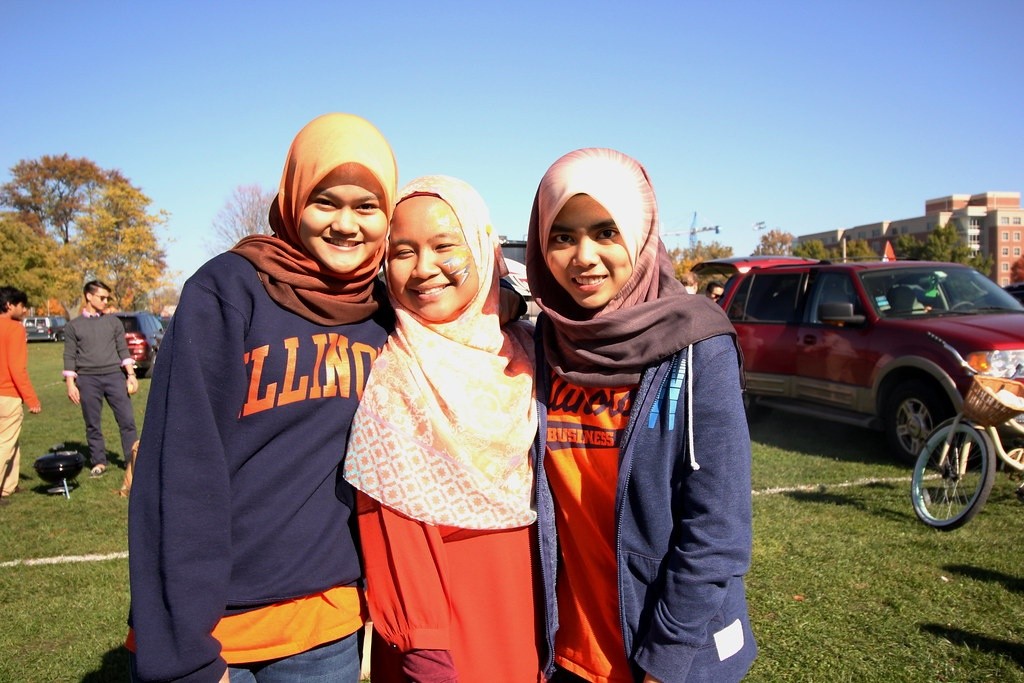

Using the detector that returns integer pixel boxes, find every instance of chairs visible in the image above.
[886,286,924,319]
[820,284,854,318]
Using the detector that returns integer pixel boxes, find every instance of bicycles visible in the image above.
[909,329,1024,530]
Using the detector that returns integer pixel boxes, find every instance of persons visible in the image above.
[706,281,724,303]
[63,280,140,476]
[680,273,699,294]
[342,174,544,683]
[526,148,758,683]
[128,113,398,683]
[0,286,42,497]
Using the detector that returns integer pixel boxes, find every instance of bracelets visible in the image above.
[127,374,136,377]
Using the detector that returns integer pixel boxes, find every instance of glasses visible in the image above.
[92,293,113,302]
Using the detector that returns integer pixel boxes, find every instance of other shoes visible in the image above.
[1,489,19,500]
[90,464,108,477]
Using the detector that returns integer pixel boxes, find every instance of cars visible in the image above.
[500,240,533,299]
[23,315,66,342]
[111,311,166,378]
[688,254,1023,470]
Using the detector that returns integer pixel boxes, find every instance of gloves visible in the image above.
[402,648,459,683]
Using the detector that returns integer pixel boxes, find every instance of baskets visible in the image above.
[964,375,1024,426]
